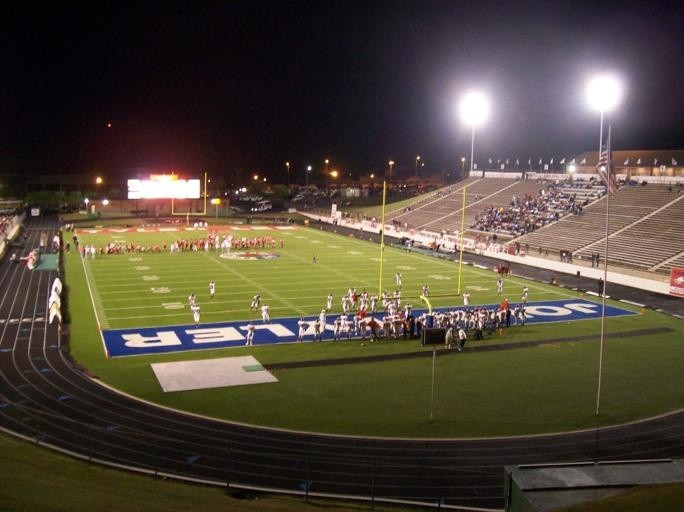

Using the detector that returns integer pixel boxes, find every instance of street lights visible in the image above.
[84,198,89,209]
[215,198,221,219]
[457,85,493,175]
[584,69,628,165]
[460,155,465,174]
[280,153,430,188]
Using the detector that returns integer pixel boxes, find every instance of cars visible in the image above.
[294,195,304,202]
[239,195,255,201]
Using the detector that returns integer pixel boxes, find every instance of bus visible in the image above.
[249,200,273,212]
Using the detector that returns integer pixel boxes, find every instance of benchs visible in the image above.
[374,176,683,276]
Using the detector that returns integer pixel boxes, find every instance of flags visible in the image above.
[595,134,619,195]
